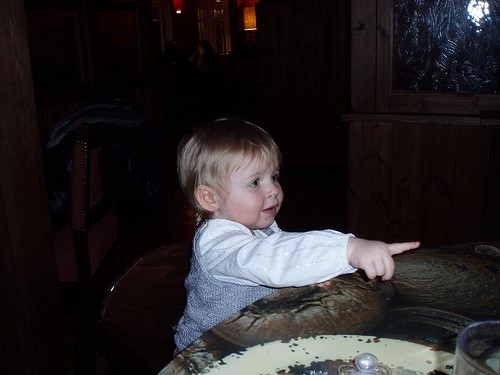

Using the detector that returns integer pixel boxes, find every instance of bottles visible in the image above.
[338,352,390,375]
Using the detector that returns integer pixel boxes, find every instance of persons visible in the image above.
[189,40,218,75]
[227,31,258,97]
[163,40,203,100]
[173,114,421,358]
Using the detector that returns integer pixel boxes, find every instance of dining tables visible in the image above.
[156,241,500,374]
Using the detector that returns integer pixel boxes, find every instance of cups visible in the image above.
[452,321,500,374]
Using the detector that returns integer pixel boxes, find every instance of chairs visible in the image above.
[97,241,191,374]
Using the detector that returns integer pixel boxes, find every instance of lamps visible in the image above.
[238,0,258,31]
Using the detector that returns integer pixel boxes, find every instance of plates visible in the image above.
[474,244,500,258]
